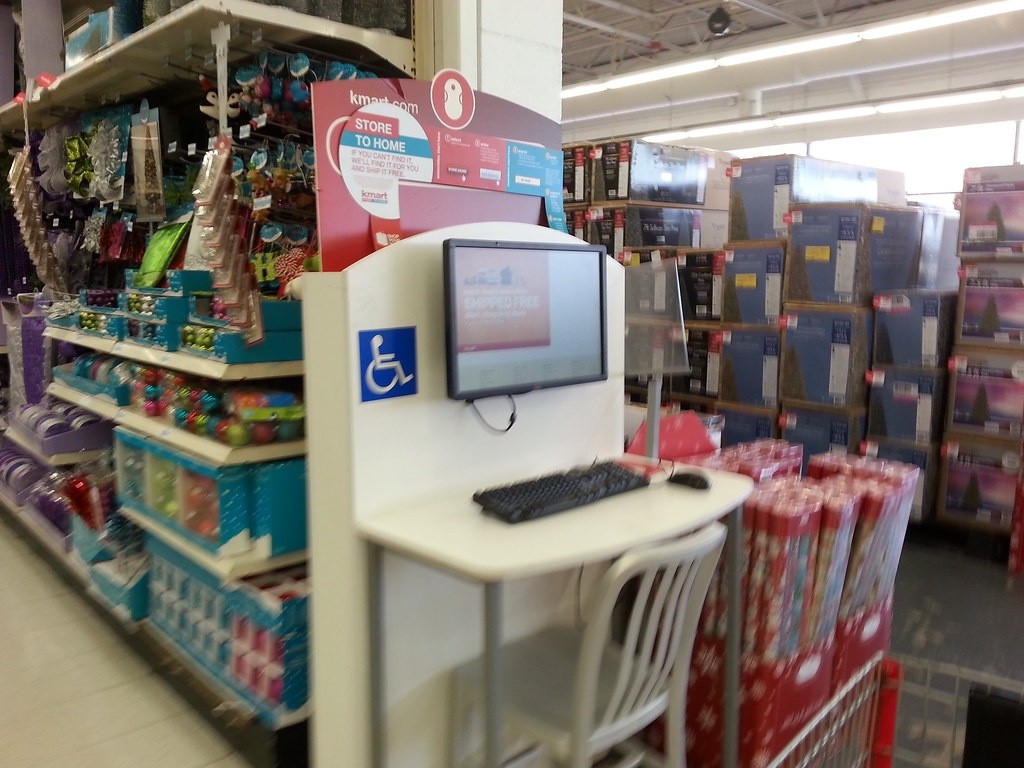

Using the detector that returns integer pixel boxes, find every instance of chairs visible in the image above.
[448,527,726,768]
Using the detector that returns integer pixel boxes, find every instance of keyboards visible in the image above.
[471,460,651,523]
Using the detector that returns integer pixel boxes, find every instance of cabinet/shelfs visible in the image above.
[0,0,434,768]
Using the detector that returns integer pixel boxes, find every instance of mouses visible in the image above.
[668,473,708,489]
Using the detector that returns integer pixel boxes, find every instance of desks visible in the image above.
[361,455,752,768]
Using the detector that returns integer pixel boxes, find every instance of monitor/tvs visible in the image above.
[442,239,607,399]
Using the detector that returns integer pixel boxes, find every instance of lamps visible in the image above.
[708,9,747,36]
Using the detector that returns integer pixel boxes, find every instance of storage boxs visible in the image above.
[873,289,955,368]
[718,326,780,410]
[116,427,307,558]
[148,538,310,712]
[716,405,775,453]
[866,435,936,526]
[675,250,721,323]
[778,303,872,410]
[868,366,946,443]
[728,153,906,243]
[65,6,126,72]
[562,138,729,263]
[722,244,783,326]
[622,245,674,316]
[937,164,1024,529]
[780,407,868,476]
[670,325,719,399]
[782,202,922,307]
[626,318,672,397]
